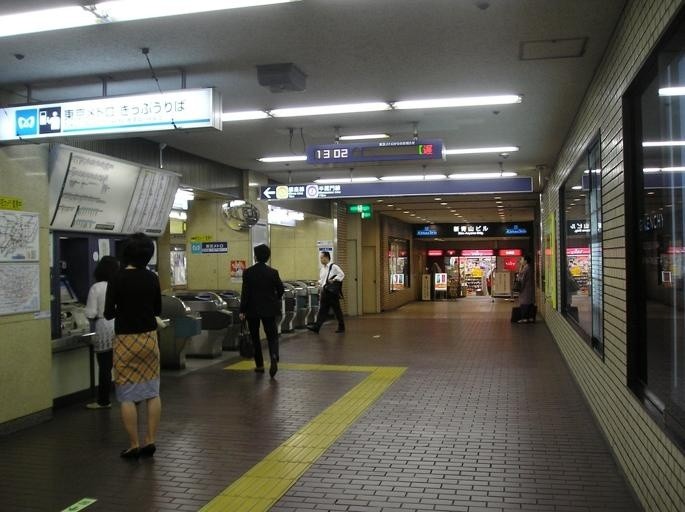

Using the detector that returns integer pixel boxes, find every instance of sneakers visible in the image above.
[85,402,111,408]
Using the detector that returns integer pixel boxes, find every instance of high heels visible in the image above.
[139,443,156,458]
[120,446,141,461]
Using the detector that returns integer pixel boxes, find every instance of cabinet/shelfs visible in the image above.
[465,275,486,296]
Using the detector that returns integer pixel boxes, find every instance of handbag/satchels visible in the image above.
[324,276,342,297]
[512,280,521,292]
[155,316,165,332]
[239,315,257,358]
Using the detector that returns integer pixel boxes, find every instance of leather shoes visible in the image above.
[270,355,277,377]
[335,329,344,333]
[254,367,265,374]
[307,326,320,333]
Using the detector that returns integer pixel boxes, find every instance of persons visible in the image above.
[569,260,589,275]
[306,251,346,333]
[83,255,114,410]
[238,243,286,377]
[103,233,162,460]
[516,255,536,323]
[454,260,496,302]
